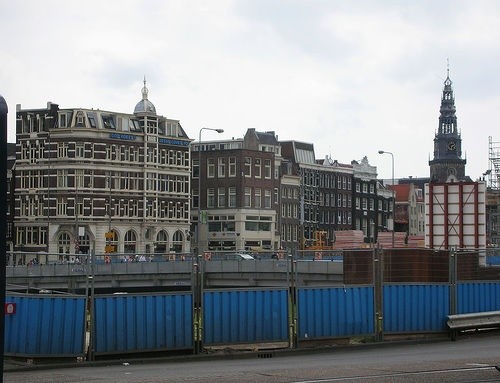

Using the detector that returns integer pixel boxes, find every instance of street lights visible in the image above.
[198,128,225,252]
[378,149,394,247]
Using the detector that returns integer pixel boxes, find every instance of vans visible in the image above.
[225,254,254,260]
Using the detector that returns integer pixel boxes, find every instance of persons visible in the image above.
[277,246,285,259]
[5,252,212,268]
[314,244,322,259]
